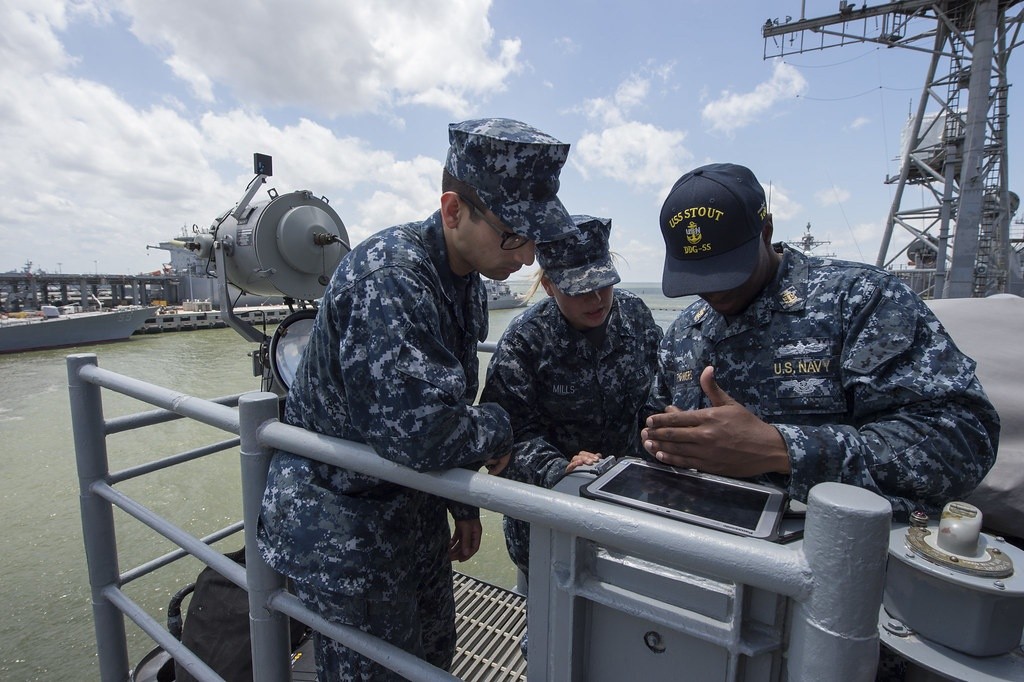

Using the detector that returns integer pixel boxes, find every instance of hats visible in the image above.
[534,216,620,295]
[659,163,766,298]
[447,119,580,242]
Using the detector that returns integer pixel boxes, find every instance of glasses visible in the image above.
[458,192,528,250]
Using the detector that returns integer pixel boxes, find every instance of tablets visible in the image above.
[580,456,789,542]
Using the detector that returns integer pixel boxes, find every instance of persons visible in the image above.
[640,162,999,513]
[479,213,666,661]
[256,120,579,682]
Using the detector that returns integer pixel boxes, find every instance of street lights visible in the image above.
[95,261,98,273]
[57,263,62,274]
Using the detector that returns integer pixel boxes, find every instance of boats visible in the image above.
[482,277,528,309]
